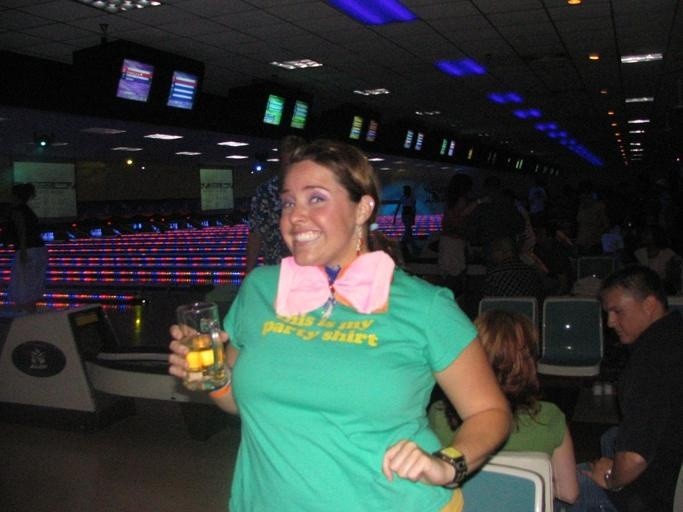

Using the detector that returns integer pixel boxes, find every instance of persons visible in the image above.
[6,180,49,316]
[168,139,512,512]
[246,135,301,269]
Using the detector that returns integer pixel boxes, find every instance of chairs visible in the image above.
[457,255,682,511]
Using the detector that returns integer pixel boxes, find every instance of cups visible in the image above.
[174,302,229,389]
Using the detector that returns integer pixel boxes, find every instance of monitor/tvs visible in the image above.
[116,58,153,106]
[263,94,560,178]
[165,69,201,112]
